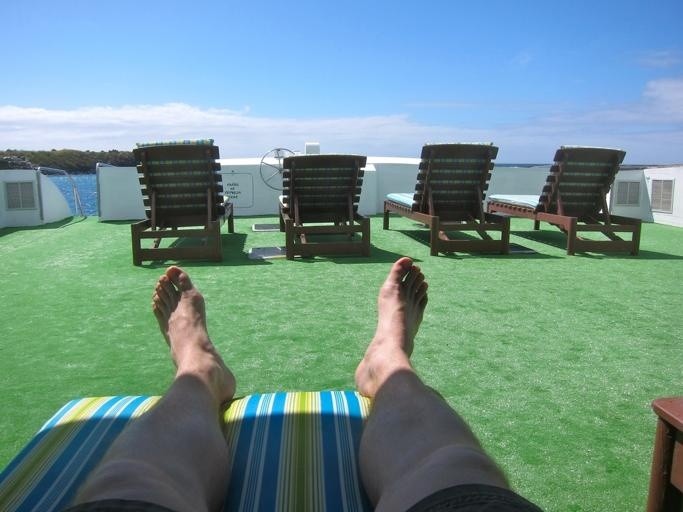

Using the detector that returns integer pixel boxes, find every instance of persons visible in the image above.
[61,254,543,511]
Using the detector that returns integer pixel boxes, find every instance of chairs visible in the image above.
[383,142,510,256]
[0,391,371,512]
[279,155,370,260]
[131,140,233,267]
[487,145,641,256]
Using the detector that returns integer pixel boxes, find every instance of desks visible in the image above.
[646,397,683,512]
